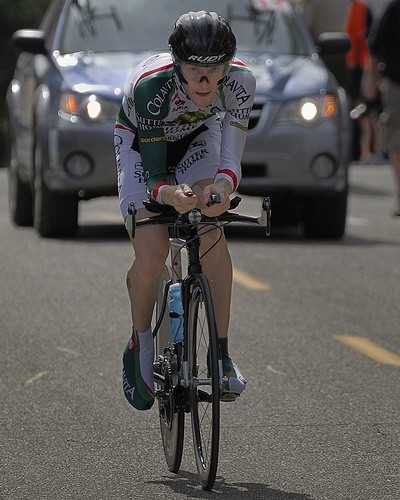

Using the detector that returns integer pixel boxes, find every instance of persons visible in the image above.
[113,10,256,411]
[360,0,400,218]
[343,0,389,161]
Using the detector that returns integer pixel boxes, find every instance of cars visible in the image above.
[0,0,353,241]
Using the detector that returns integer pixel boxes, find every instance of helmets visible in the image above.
[169,11,236,65]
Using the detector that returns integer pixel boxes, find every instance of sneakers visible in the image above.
[122,327,154,410]
[206,349,246,393]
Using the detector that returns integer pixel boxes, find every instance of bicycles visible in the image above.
[125,192,274,492]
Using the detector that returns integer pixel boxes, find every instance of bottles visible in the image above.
[168,283,184,342]
